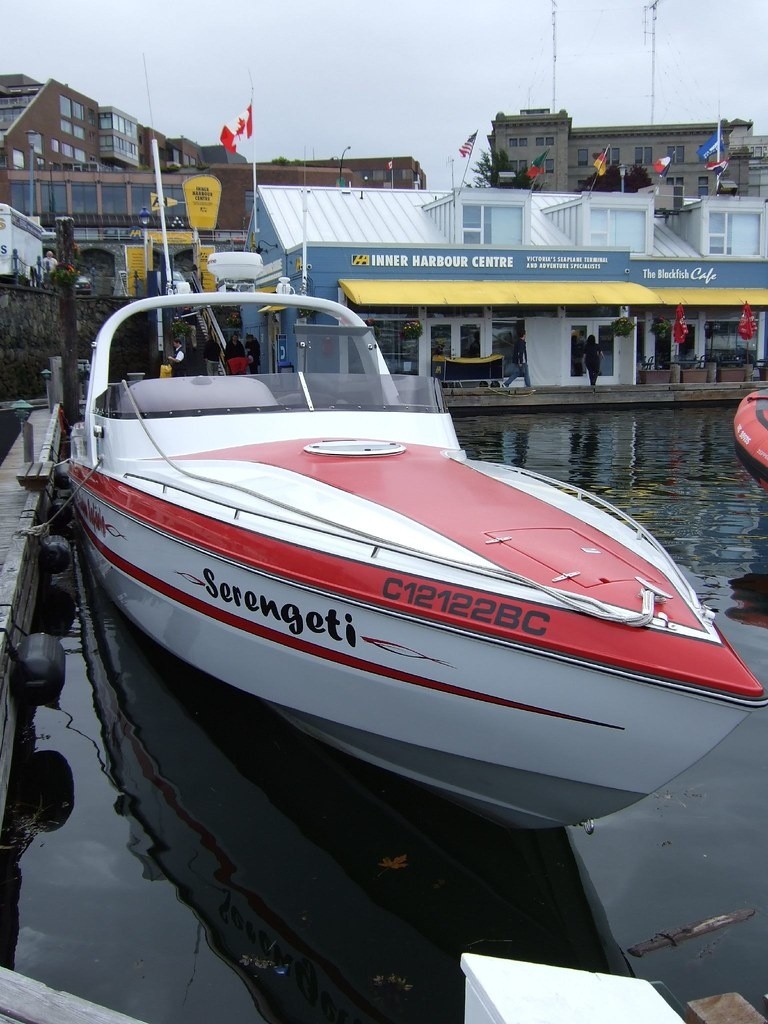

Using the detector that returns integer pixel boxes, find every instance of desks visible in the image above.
[663,360,699,369]
[719,359,742,367]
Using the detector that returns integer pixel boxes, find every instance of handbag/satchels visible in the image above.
[159,358,172,378]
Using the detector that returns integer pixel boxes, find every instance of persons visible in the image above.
[502,329,530,389]
[167,339,186,378]
[582,335,606,386]
[206,328,260,376]
[43,251,59,283]
[183,308,199,352]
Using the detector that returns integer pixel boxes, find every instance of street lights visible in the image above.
[139,206,151,278]
[616,164,627,192]
[329,145,351,183]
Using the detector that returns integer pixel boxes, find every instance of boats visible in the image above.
[53,49,768,835]
[733,387,768,479]
[66,532,636,1024]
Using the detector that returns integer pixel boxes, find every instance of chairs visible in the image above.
[749,353,767,369]
[638,352,744,368]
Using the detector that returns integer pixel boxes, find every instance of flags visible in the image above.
[696,120,724,164]
[653,155,673,178]
[220,99,253,156]
[525,166,545,179]
[704,158,732,180]
[531,148,551,169]
[386,161,395,171]
[594,152,606,176]
[459,130,480,157]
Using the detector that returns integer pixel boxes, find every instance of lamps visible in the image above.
[255,239,278,254]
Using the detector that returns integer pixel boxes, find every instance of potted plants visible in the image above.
[757,363,768,381]
[716,364,747,382]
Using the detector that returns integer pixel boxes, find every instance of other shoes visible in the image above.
[502,383,507,388]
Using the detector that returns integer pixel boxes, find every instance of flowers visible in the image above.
[610,316,638,340]
[648,316,673,338]
[51,263,82,288]
[399,320,424,341]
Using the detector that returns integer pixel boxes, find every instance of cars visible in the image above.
[154,269,186,295]
[75,275,91,296]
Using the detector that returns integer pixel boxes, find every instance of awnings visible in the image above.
[341,279,768,305]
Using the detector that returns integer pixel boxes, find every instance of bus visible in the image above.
[0,203,46,282]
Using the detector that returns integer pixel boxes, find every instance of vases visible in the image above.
[639,369,672,384]
[680,369,708,383]
